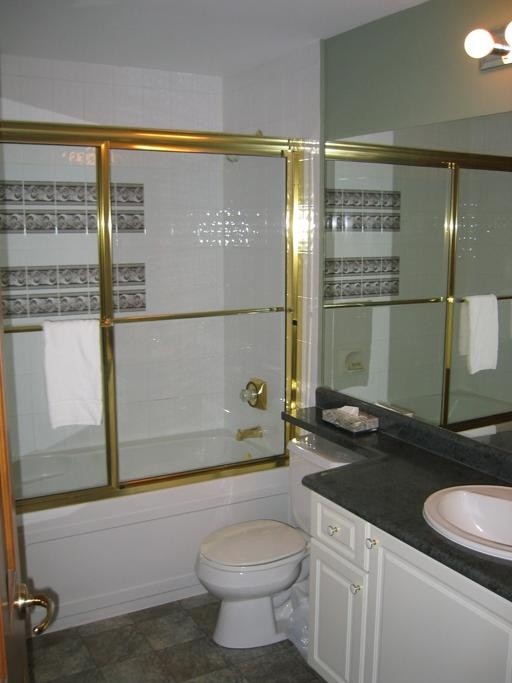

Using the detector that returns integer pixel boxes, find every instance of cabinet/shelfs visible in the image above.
[307,489,512,682]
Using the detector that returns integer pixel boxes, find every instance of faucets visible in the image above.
[236,427,263,442]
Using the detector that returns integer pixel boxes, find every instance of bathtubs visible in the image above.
[9,426,304,636]
[388,391,512,426]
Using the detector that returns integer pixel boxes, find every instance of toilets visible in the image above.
[195,432,368,651]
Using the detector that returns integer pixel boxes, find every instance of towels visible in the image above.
[43,318,105,430]
[458,294,500,376]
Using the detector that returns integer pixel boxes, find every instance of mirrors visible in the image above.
[319,104,510,486]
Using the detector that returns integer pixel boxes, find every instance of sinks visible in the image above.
[423,484,512,562]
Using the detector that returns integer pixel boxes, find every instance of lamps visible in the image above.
[464,20,512,70]
